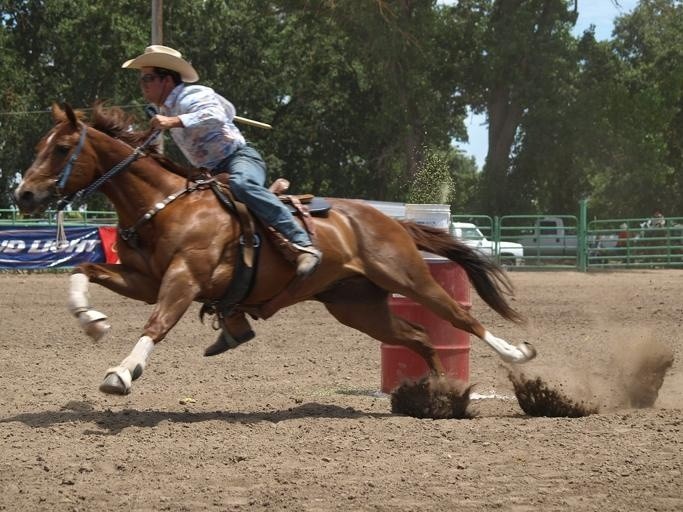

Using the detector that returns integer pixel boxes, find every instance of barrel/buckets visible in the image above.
[404,203,452,260]
[380,258,472,395]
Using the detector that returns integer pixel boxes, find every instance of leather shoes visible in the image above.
[204,331,256,357]
[295,245,323,283]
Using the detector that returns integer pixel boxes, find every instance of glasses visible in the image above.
[140,73,166,82]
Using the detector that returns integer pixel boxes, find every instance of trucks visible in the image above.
[363,198,523,271]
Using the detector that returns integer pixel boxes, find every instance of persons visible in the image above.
[119,42,323,360]
[614,208,683,267]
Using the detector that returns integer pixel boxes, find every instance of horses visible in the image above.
[12,98,538,396]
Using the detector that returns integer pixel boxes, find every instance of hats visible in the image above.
[122,45,200,83]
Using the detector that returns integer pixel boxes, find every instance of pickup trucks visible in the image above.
[515,214,626,259]
